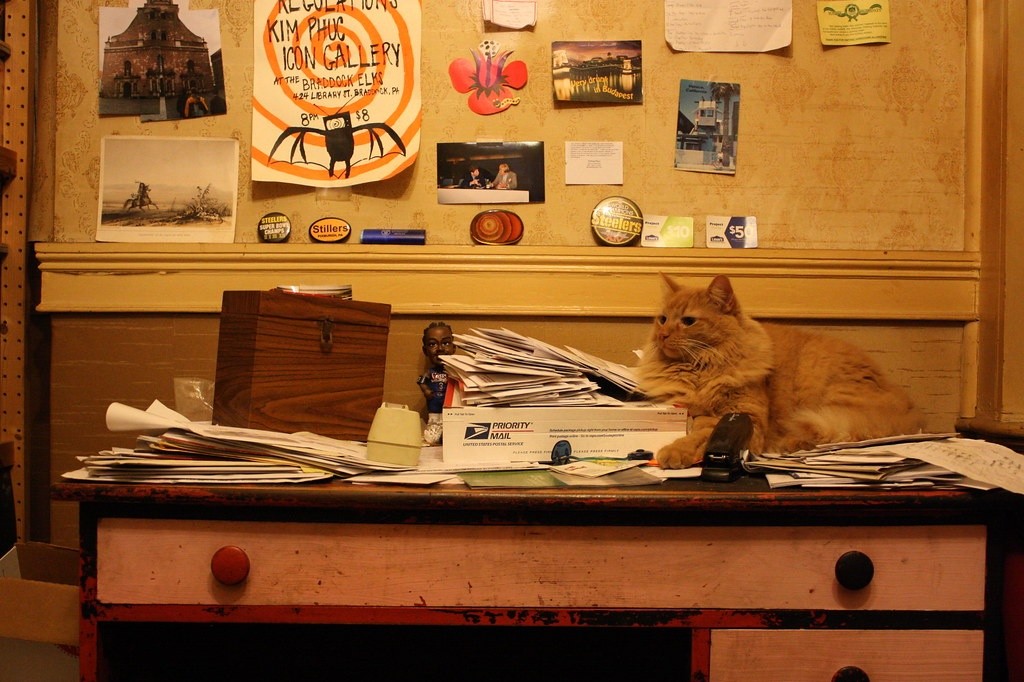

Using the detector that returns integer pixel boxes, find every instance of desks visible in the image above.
[52,455,1018,682]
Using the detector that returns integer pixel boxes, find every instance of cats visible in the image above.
[635,271,956,470]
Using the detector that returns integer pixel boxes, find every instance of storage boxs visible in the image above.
[0,542,81,648]
[211,291,391,442]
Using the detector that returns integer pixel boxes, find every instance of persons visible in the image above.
[460,163,518,190]
[417,321,456,445]
[178,86,225,119]
[716,150,723,168]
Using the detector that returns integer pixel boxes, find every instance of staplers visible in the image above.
[698,411,755,485]
